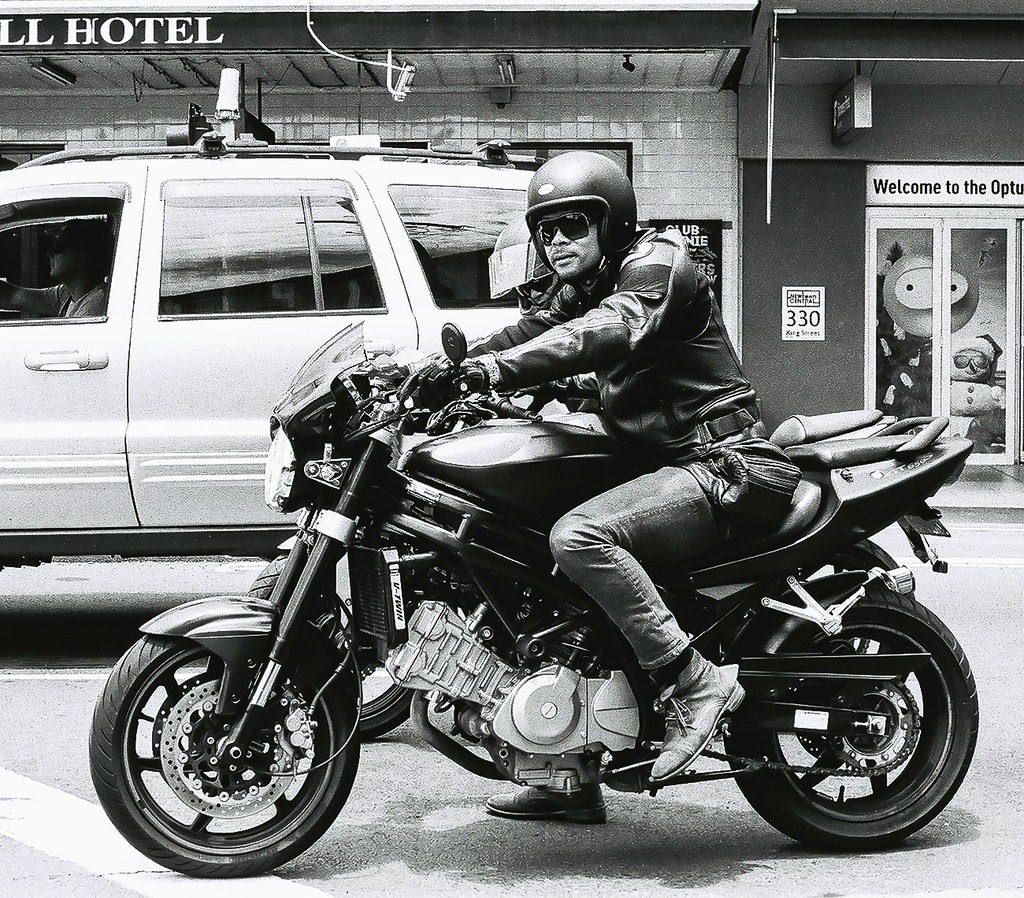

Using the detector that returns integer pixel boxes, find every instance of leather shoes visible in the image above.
[650,647,746,782]
[486,783,606,823]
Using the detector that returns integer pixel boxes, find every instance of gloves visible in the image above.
[395,353,489,412]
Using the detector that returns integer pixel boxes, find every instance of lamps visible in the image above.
[26,58,77,88]
[496,52,515,84]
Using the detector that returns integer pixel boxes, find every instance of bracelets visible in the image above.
[474,354,501,384]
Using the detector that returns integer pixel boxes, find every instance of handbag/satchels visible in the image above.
[676,437,804,524]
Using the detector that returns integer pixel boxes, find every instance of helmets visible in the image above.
[525,151,637,271]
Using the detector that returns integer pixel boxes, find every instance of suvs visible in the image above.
[0,134,650,629]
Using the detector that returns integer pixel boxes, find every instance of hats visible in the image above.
[45,217,113,261]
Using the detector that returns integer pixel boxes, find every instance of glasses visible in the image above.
[536,211,602,246]
[51,240,74,254]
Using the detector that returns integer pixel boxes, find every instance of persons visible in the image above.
[0,219,115,319]
[363,149,769,825]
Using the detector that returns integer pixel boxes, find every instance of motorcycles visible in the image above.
[85,315,982,879]
